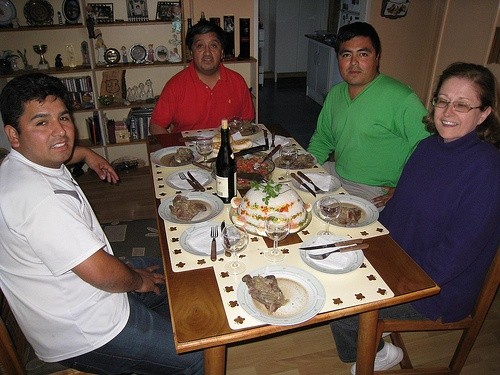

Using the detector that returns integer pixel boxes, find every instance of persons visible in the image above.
[306,21,437,222]
[323,56,500,374]
[0,73,212,375]
[150,21,257,135]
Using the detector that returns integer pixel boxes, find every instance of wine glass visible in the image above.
[264,213,291,262]
[278,145,298,182]
[197,137,214,167]
[221,224,249,275]
[317,196,342,236]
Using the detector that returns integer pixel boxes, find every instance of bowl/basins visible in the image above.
[98,94,115,105]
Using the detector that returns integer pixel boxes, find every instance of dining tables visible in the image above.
[145,125,440,375]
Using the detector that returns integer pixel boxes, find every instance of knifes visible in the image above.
[220,220,232,257]
[264,131,270,149]
[298,239,363,250]
[291,172,316,198]
[187,171,205,192]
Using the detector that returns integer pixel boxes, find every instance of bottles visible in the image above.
[130,119,137,140]
[215,119,238,206]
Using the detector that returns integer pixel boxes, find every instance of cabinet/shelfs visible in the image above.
[306,39,344,106]
[0,0,259,176]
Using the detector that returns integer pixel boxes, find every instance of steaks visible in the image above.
[174,147,193,163]
[337,207,361,225]
[168,194,207,220]
[242,274,283,313]
[280,153,315,169]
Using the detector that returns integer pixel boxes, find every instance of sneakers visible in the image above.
[351,342,404,375]
[382,331,392,338]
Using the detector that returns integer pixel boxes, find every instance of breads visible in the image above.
[210,133,252,153]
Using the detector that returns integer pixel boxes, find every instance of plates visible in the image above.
[238,265,327,326]
[150,123,379,256]
[104,43,169,64]
[0,0,81,27]
[300,234,363,274]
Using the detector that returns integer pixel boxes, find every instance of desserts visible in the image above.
[231,177,312,236]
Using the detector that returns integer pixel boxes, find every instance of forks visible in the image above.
[297,171,326,193]
[210,226,218,261]
[178,172,200,191]
[309,243,370,259]
[271,132,276,147]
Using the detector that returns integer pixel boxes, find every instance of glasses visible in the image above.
[431,97,488,113]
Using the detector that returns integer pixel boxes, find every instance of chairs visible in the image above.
[372,240,500,375]
[0,288,89,375]
